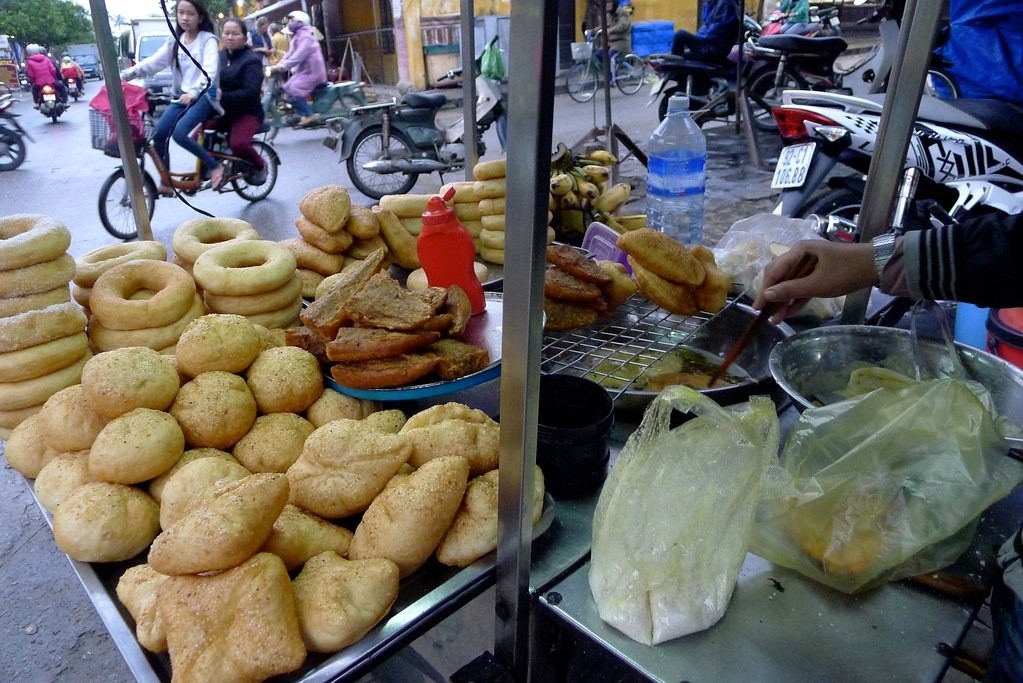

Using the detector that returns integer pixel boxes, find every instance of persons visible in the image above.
[754,209,1023,324]
[25,44,87,110]
[277,11,327,125]
[671,0,742,63]
[121,0,224,195]
[585,0,632,87]
[777,0,809,35]
[191,18,267,184]
[244,15,297,93]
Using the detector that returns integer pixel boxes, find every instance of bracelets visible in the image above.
[872,233,895,288]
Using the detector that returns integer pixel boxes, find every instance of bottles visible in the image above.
[647,95,705,243]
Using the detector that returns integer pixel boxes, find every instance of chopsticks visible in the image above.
[706,251,816,388]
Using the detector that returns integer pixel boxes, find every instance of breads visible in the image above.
[277,184,418,297]
[4,313,546,683]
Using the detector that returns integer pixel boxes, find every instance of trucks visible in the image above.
[117,18,175,116]
[67,44,102,80]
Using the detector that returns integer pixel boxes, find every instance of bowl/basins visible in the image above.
[769,326,1022,451]
[318,290,546,418]
[541,302,796,419]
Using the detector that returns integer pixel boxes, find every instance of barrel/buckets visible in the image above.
[984,306,1023,373]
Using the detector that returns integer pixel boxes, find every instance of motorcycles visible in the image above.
[32,84,72,123]
[17,73,32,92]
[65,78,85,102]
[0,81,35,171]
[649,14,765,128]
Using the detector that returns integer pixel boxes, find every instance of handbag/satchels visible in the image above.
[91,79,150,147]
[480,41,505,81]
[586,384,781,646]
[693,212,853,321]
[744,356,1023,595]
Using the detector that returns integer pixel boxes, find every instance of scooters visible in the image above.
[258,66,367,143]
[739,0,1023,242]
[326,35,508,200]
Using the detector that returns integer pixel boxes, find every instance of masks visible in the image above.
[288,23,297,32]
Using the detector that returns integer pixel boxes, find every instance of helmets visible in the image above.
[62,52,71,62]
[287,11,311,25]
[26,44,47,55]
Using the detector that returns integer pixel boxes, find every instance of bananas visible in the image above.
[546,142,647,235]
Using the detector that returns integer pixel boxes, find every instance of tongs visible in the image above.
[912,297,971,392]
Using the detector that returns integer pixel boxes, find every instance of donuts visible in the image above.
[381,156,555,290]
[0,212,304,443]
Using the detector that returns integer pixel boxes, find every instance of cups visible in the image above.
[955,302,991,352]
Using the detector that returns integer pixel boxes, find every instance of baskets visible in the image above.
[90,108,154,155]
[571,42,594,59]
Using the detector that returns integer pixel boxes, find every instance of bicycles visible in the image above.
[566,30,646,102]
[98,82,281,241]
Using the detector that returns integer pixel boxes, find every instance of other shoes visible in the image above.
[144,184,175,198]
[279,103,295,110]
[213,165,228,190]
[255,161,269,185]
[299,114,316,125]
